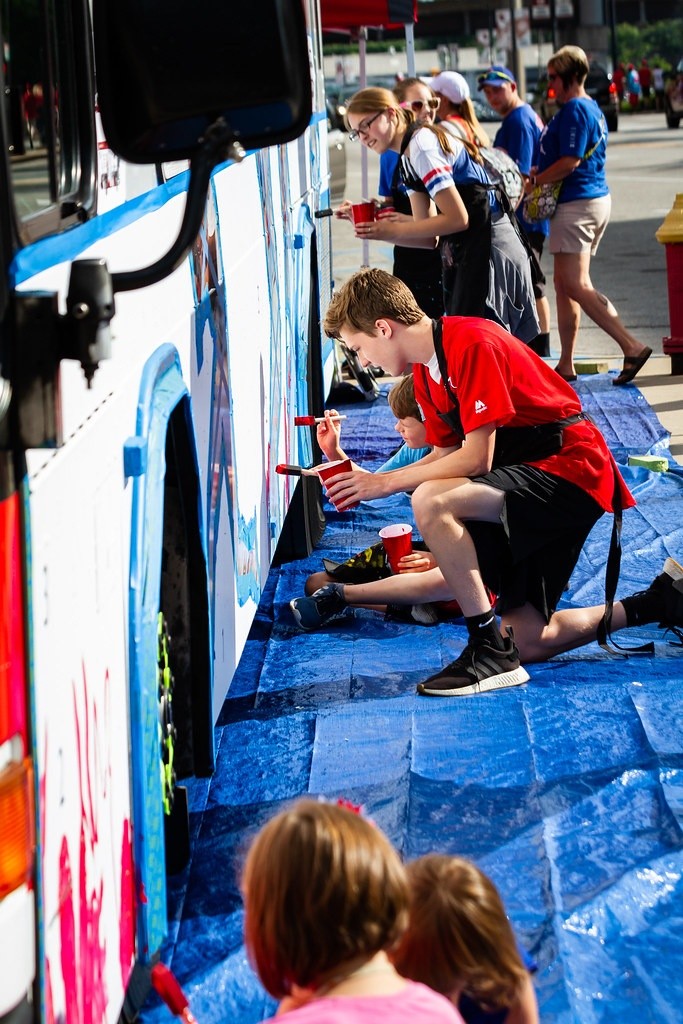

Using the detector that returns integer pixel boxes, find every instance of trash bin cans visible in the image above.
[655,193,683,377]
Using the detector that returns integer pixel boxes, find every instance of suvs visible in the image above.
[661,58,683,129]
[528,59,619,132]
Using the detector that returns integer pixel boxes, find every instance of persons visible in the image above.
[336,45,654,385]
[242,798,539,1024]
[291,269,683,697]
[613,60,664,111]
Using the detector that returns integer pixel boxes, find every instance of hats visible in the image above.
[477,66,514,90]
[419,71,470,105]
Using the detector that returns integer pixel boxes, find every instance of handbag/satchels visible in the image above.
[524,179,563,220]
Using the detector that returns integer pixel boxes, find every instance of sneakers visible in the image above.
[387,602,438,626]
[290,585,346,632]
[417,625,530,695]
[646,556,683,643]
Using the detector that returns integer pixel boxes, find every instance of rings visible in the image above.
[369,228,370,232]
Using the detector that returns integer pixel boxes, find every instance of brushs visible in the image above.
[294,415,348,426]
[275,464,318,477]
[359,197,385,209]
[315,206,350,218]
[153,964,199,1024]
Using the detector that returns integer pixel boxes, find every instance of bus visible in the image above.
[0,0,333,1024]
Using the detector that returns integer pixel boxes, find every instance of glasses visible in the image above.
[348,106,384,142]
[548,72,562,83]
[477,71,513,83]
[399,97,441,113]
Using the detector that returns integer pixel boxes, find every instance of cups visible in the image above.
[350,201,375,235]
[379,523,412,576]
[374,207,396,220]
[315,458,361,513]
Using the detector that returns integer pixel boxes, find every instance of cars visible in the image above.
[323,68,505,134]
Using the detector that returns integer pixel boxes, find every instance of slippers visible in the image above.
[617,348,652,384]
[552,368,579,382]
